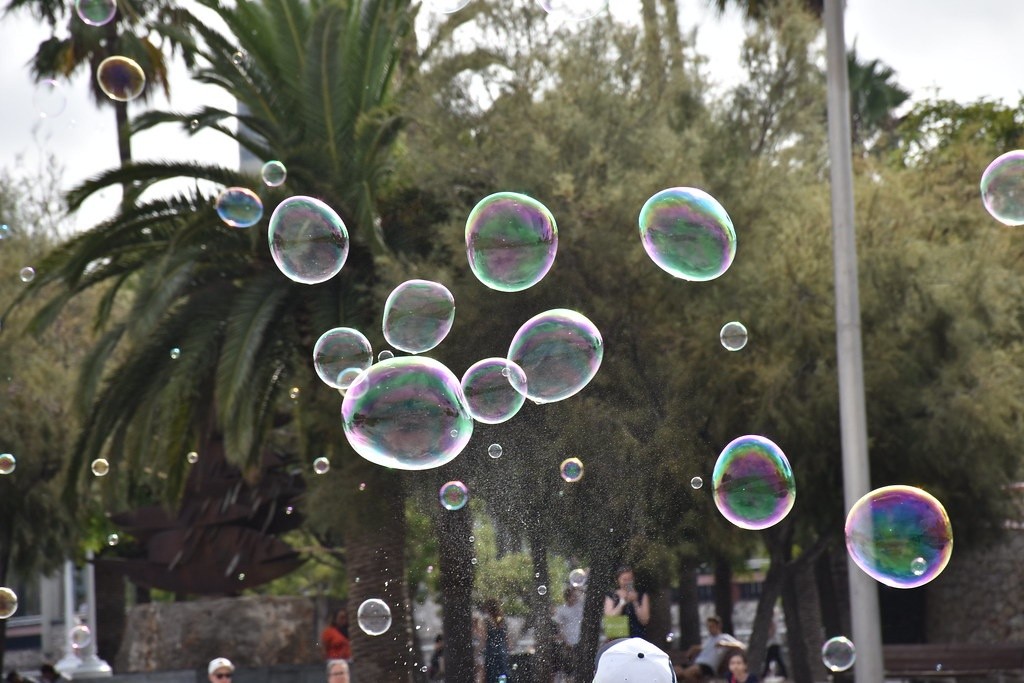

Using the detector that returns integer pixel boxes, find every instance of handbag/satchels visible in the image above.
[603,595,630,639]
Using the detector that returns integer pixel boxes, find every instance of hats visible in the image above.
[208,657,234,674]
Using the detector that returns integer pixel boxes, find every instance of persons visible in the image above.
[7,664,70,683]
[208,563,789,683]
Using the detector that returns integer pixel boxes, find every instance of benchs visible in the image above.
[881,642,1024,683]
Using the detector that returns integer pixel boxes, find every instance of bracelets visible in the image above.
[620,599,627,606]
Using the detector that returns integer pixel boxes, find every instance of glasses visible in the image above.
[329,671,349,676]
[212,673,233,678]
